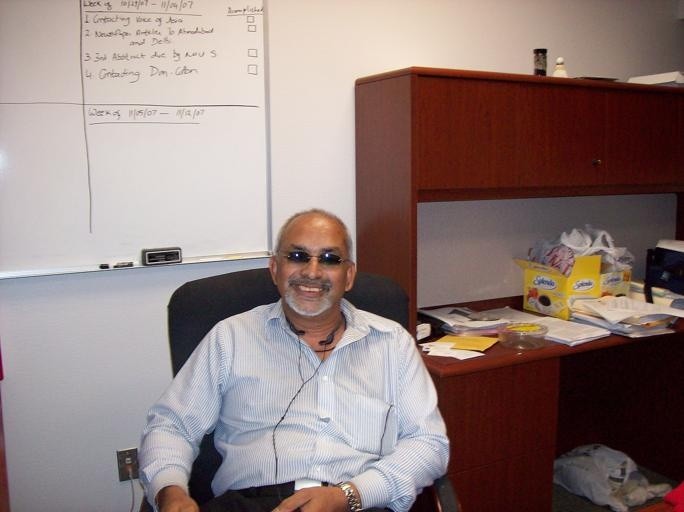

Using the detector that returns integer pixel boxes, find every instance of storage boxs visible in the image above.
[513,248,633,320]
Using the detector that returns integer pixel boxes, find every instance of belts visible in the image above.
[238,479,335,494]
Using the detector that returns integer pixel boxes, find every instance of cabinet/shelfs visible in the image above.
[356,67,684,512]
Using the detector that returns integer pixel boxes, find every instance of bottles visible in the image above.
[532,47,548,75]
[551,56,568,76]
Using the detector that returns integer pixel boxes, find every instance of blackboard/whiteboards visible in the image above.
[0,0,274,280]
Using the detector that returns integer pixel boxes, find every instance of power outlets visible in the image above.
[117,448,138,481]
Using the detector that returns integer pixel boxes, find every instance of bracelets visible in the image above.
[335,481,362,512]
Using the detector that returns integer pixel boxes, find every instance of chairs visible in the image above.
[139,268,458,511]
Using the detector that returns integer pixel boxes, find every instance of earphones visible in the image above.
[290,324,304,335]
[319,333,334,345]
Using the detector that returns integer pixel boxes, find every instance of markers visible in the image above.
[99,262,134,269]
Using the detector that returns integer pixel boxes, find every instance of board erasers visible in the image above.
[142,247,182,266]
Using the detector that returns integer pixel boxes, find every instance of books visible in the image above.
[419,280,684,360]
[622,71,683,87]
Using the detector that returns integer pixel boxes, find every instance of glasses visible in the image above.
[277,252,350,269]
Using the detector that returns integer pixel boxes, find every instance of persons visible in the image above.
[138,209,450,512]
